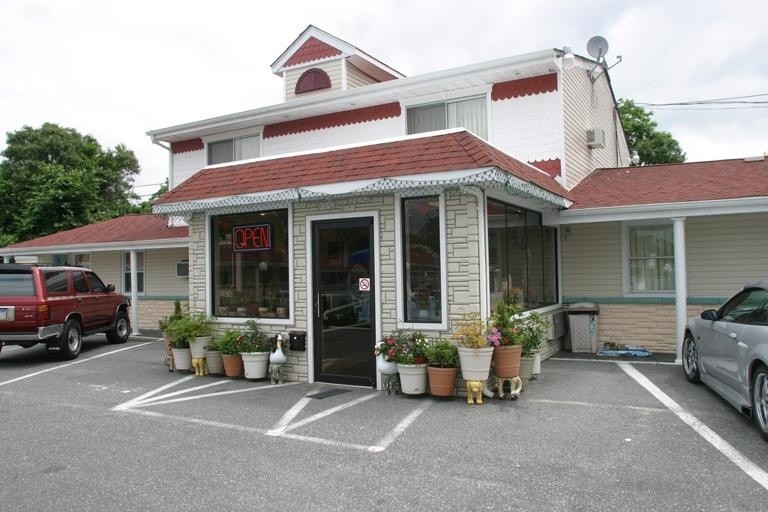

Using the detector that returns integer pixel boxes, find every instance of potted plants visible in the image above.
[427,339,460,396]
[526,309,553,375]
[218,287,286,317]
[269,334,287,363]
[218,328,243,376]
[164,315,192,371]
[490,303,526,377]
[519,328,538,378]
[236,319,270,378]
[205,338,223,373]
[187,311,213,359]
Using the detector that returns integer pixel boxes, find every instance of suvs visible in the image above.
[0,264,131,359]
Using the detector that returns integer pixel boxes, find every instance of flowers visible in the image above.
[159,319,173,355]
[400,329,428,364]
[375,337,399,360]
[448,309,502,348]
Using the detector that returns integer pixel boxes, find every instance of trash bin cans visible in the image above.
[567,302,600,354]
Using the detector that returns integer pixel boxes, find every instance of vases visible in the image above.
[456,346,495,381]
[397,364,427,394]
[377,354,398,374]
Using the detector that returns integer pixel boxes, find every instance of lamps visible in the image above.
[556,47,575,60]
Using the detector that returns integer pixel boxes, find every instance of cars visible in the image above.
[682,282,768,441]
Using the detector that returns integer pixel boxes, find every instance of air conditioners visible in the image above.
[586,128,605,148]
